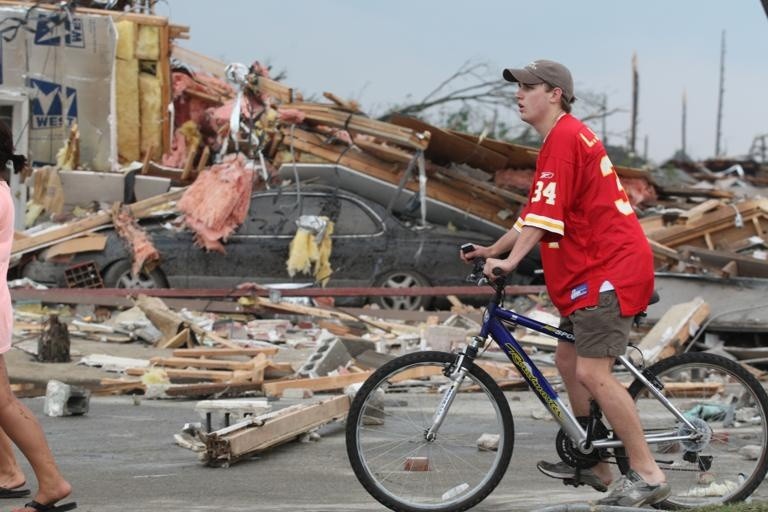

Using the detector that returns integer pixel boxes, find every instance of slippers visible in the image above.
[1,487,32,498]
[25,501,77,512]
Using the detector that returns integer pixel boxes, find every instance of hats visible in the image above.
[504,61,575,105]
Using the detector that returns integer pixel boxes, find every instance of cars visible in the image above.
[14,179,526,316]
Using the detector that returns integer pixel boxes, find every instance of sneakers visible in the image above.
[538,459,608,493]
[592,469,671,512]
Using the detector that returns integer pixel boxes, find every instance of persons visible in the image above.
[460,60,672,507]
[0,124,79,512]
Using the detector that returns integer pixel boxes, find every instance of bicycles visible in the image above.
[341,250,768,511]
